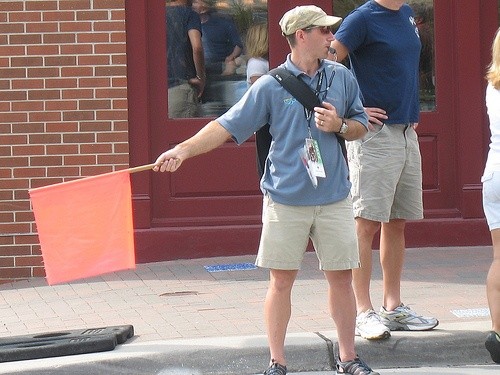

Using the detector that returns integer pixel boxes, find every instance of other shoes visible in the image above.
[484,331,500,363]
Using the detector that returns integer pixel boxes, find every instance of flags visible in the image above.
[27,169,135,285]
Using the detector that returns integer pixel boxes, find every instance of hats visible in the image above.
[279,4,347,39]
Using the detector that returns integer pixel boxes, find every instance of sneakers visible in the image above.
[379,306,440,331]
[354,309,391,340]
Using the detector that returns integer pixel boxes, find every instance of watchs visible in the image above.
[336,116,348,134]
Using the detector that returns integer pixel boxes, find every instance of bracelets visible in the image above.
[195,74,202,79]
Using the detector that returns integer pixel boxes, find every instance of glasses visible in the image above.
[305,25,332,35]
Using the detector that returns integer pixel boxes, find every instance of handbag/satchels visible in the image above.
[255,68,350,180]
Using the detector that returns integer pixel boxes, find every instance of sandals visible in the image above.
[336,355,380,375]
[263,359,287,375]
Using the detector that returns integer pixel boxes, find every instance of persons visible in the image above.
[163,0,207,117]
[246,25,270,90]
[411,11,434,95]
[190,0,243,117]
[321,0,439,340]
[480,28,499,364]
[152,5,382,374]
